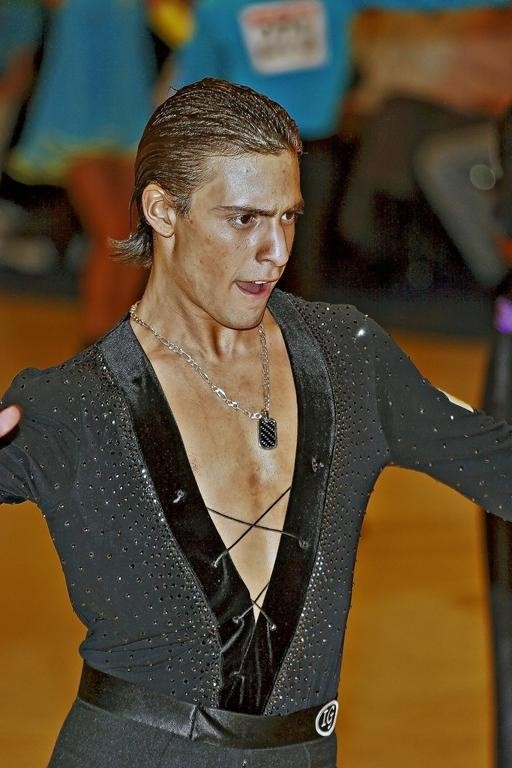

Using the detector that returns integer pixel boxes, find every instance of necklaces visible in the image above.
[127,293,279,452]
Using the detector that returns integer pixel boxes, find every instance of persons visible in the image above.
[0,0,200,359]
[1,2,512,313]
[1,76,511,766]
